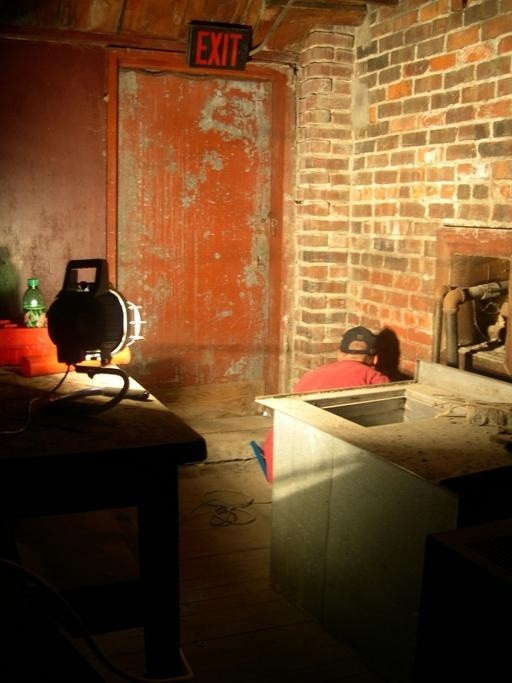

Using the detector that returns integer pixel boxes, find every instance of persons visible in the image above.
[263,325,391,486]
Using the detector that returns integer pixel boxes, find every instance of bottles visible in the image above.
[22,276,46,328]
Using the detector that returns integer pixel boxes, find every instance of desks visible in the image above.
[0,360,208,681]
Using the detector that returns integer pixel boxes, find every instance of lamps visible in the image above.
[41,257,150,416]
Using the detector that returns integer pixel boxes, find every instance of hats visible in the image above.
[341,325,381,356]
